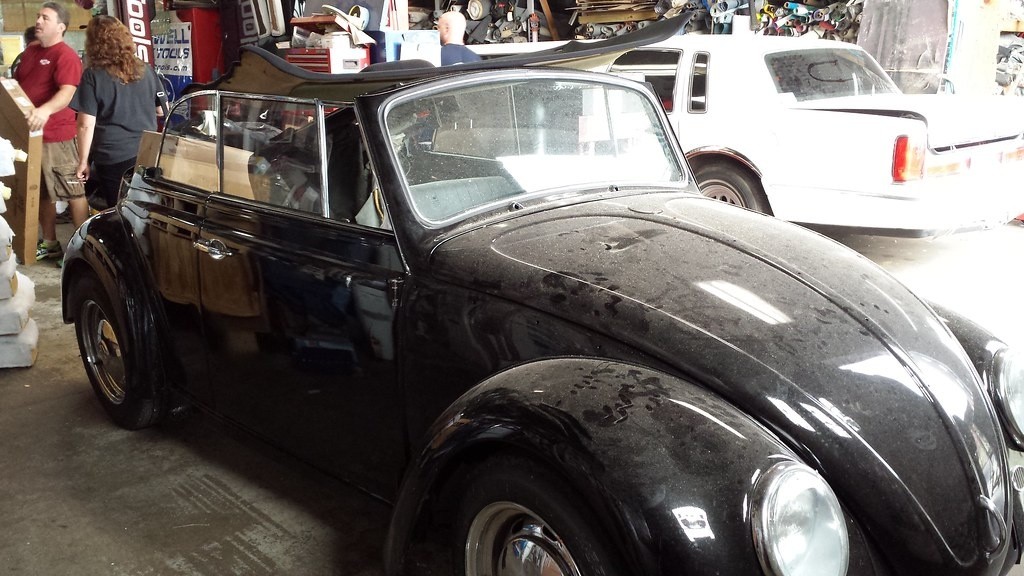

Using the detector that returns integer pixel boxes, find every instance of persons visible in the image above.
[433,11,482,67]
[10,2,170,262]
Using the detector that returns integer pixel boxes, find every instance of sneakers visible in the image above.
[35,239,62,259]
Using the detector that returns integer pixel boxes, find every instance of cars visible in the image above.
[414,30,1024,246]
[59,58,1024,576]
